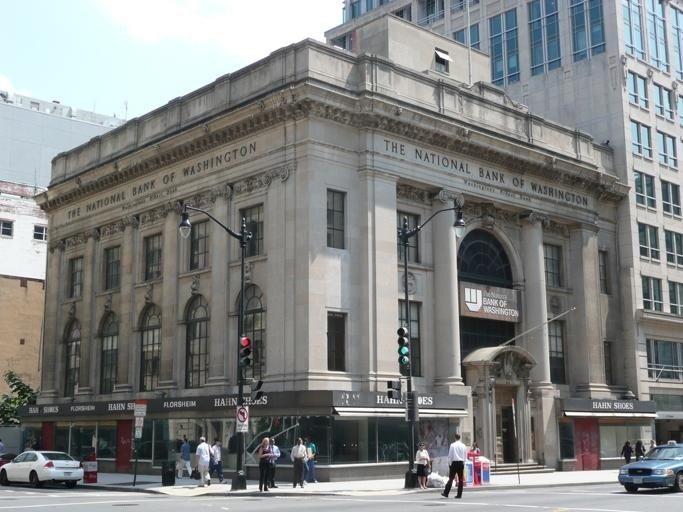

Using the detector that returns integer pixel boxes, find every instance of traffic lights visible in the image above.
[396,327,410,376]
[250,379,265,402]
[239,334,251,368]
[386,379,402,400]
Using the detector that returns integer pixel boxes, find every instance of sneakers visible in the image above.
[197,479,227,487]
[441,492,461,498]
[293,480,318,488]
[259,485,278,491]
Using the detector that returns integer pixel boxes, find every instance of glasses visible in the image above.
[419,444,424,447]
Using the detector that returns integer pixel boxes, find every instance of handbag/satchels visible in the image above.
[191,469,201,480]
[175,459,185,471]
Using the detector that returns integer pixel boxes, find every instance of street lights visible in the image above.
[177,203,249,493]
[402,201,468,489]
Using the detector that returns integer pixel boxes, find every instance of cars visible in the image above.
[131,437,175,467]
[0,450,85,488]
[617,439,682,493]
[0,452,19,467]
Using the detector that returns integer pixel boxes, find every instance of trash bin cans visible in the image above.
[161,461,176,486]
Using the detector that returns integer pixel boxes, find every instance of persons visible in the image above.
[269,438,282,488]
[648,440,657,457]
[470,438,480,454]
[304,437,318,484]
[176,438,193,478]
[621,440,634,464]
[635,440,646,462]
[258,437,274,491]
[442,433,467,498]
[209,438,228,484]
[194,436,215,487]
[415,442,430,491]
[0,438,5,455]
[290,436,307,488]
